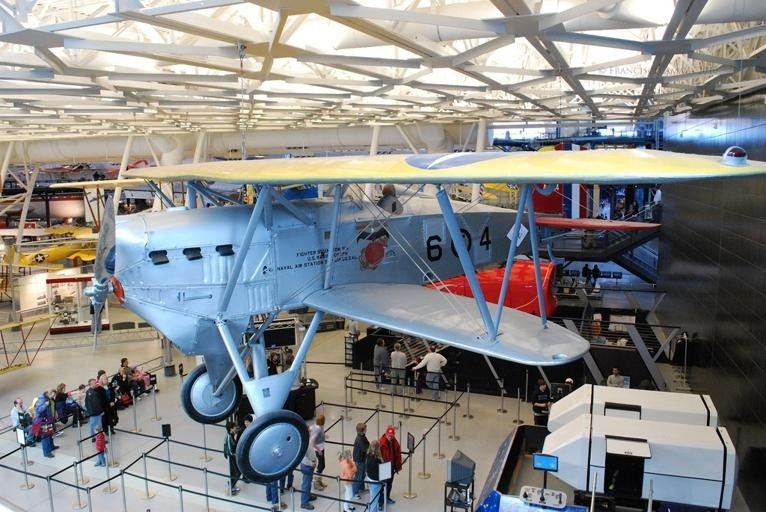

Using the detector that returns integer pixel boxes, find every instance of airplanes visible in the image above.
[1,159,151,190]
[46,138,764,485]
[3,222,100,272]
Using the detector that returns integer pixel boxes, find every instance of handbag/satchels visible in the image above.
[32,417,57,437]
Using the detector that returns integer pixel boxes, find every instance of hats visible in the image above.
[386,427,394,435]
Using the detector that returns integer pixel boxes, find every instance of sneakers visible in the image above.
[26,440,36,446]
[344,493,395,512]
[55,432,63,436]
[44,452,54,458]
[49,446,59,451]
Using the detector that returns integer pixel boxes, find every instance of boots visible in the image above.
[301,476,327,509]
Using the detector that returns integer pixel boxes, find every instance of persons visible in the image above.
[607,366,624,387]
[345,319,360,339]
[651,185,661,222]
[583,263,596,282]
[532,379,549,425]
[617,200,624,218]
[631,200,639,215]
[592,264,599,285]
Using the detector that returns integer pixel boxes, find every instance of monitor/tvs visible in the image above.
[533,453,558,471]
[447,450,476,483]
[551,383,571,400]
[624,377,630,388]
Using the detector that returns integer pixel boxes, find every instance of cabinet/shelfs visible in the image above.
[344,333,358,366]
[443,478,474,512]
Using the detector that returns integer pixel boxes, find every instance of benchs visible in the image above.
[16,371,152,442]
